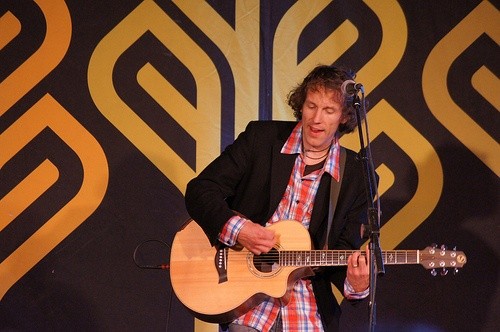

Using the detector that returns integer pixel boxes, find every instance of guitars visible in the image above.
[170,210,467,323]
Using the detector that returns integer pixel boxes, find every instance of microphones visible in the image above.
[341,79,363,97]
